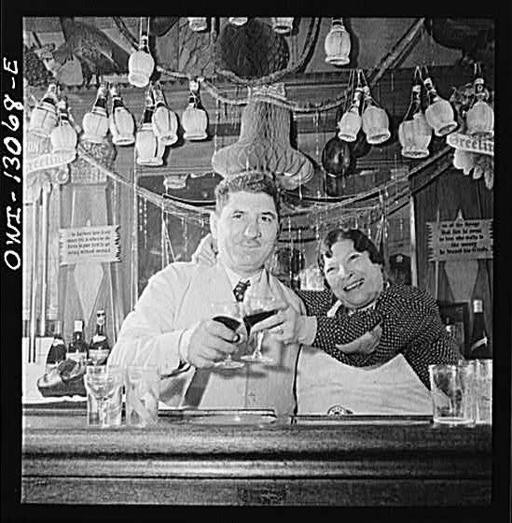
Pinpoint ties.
[233,280,252,302]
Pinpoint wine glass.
[202,298,282,370]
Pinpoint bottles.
[468,296,489,361]
[44,310,111,372]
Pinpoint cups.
[427,357,492,428]
[83,363,160,429]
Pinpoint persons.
[106,170,311,416]
[191,229,464,415]
